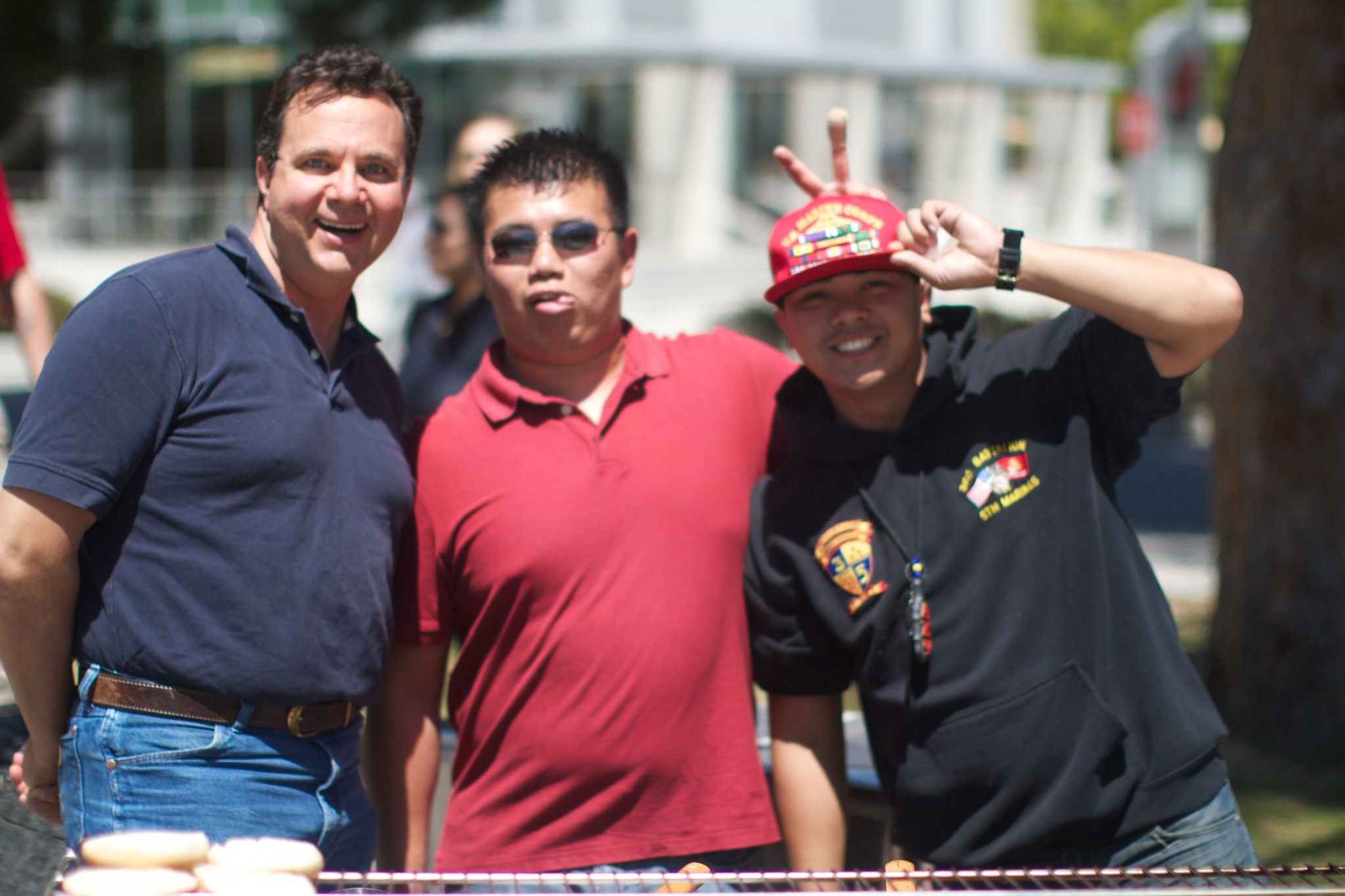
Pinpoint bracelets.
[995,228,1025,290]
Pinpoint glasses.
[473,218,622,259]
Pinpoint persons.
[0,45,423,895]
[739,194,1263,886]
[365,111,883,892]
[0,112,529,544]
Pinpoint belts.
[95,675,360,738]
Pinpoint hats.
[764,194,917,309]
